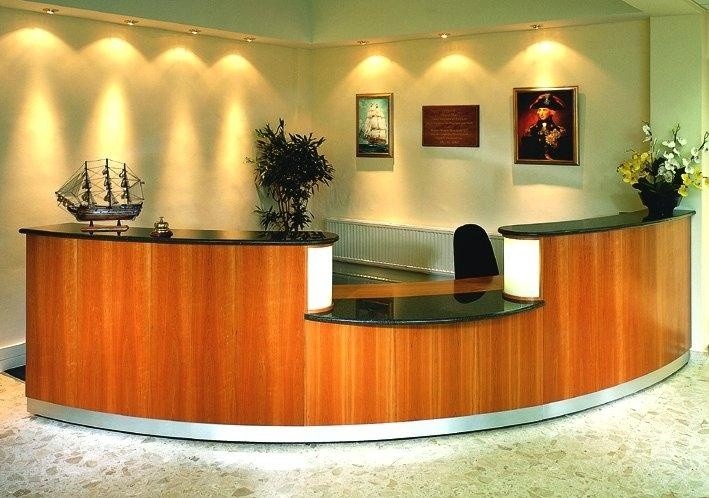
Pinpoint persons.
[521,93,572,160]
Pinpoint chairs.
[452,222,500,280]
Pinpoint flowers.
[618,119,709,197]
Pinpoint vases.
[637,190,683,218]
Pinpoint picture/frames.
[353,93,395,159]
[513,87,581,166]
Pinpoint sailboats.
[362,101,388,146]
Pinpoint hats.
[530,94,566,111]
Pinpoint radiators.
[325,216,504,278]
